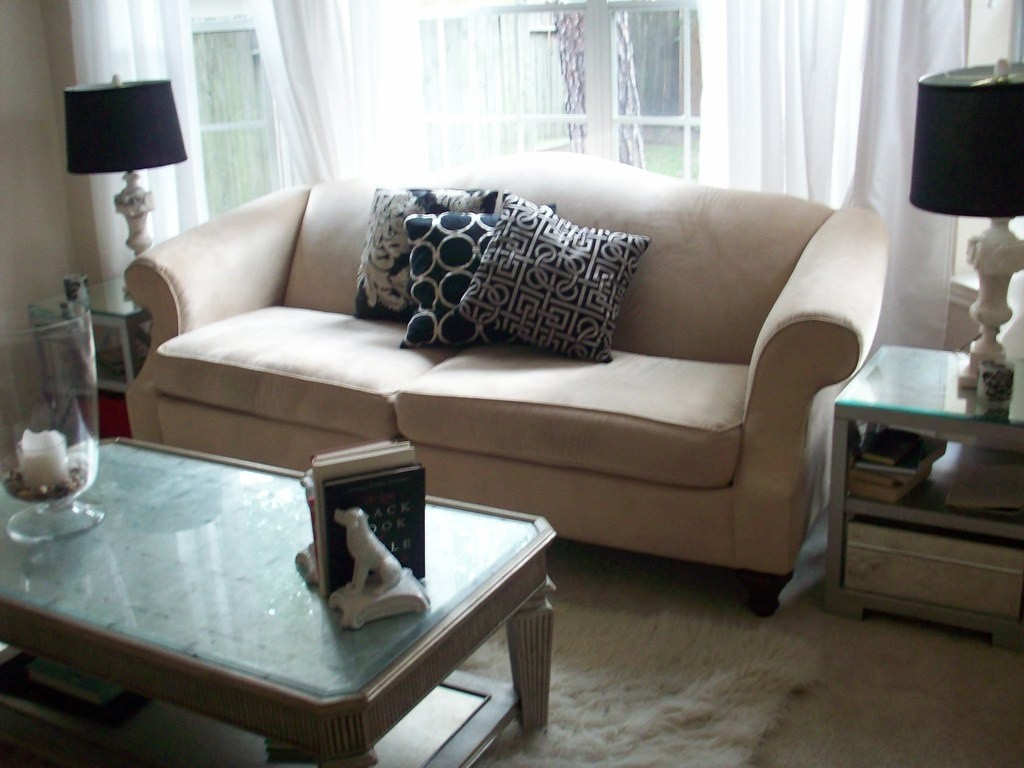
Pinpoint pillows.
[353,189,499,322]
[399,204,557,348]
[461,193,651,363]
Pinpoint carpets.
[462,605,830,768]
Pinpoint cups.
[975,359,1015,403]
[64,273,90,304]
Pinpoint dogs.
[334,507,402,597]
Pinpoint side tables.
[826,345,1024,649]
[28,277,153,393]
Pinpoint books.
[311,441,426,597]
[845,427,947,524]
[28,656,122,705]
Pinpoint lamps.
[909,65,1023,387]
[63,74,188,296]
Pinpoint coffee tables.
[0,437,559,768]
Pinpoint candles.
[20,428,66,486]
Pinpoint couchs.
[127,154,889,617]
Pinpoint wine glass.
[0,305,106,544]
